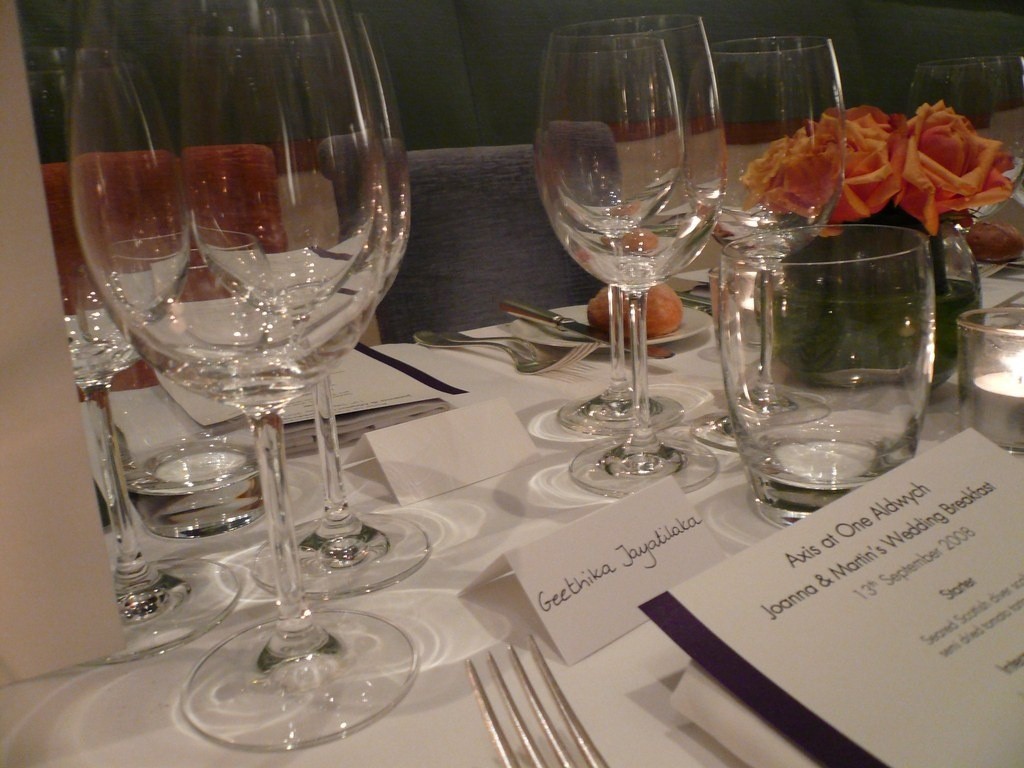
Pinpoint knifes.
[501,299,674,359]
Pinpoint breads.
[584,279,684,338]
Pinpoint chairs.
[39,145,287,399]
[313,120,632,349]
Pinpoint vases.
[753,200,984,391]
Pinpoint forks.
[415,332,601,375]
[466,634,610,768]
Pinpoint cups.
[718,226,935,528]
[957,306,1024,454]
[105,362,281,542]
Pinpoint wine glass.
[72,0,418,753]
[692,37,848,452]
[181,9,410,599]
[552,38,687,434]
[535,15,728,497]
[24,46,239,665]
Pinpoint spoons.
[437,330,610,361]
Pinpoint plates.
[512,306,713,346]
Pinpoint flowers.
[739,99,1016,241]
